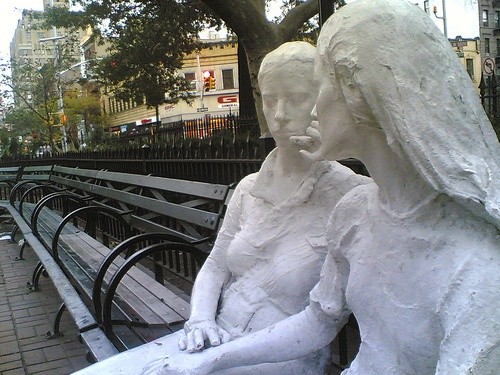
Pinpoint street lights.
[53,56,119,155]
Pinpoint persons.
[71,42,374,374]
[141,0,500,375]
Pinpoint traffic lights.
[204,77,210,92]
[210,76,215,90]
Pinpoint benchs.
[0,164,349,375]
